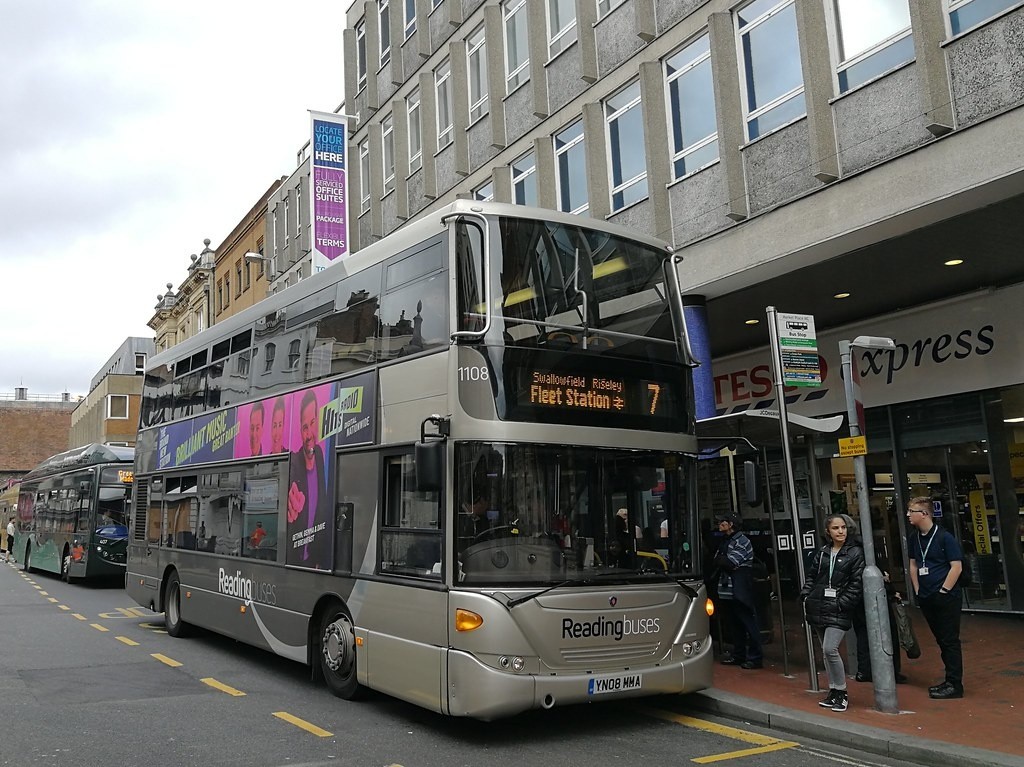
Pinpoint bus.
[124,196,715,722]
[12,443,137,582]
[0,483,22,553]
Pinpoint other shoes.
[894,674,908,683]
[855,672,872,681]
[3,559,9,564]
[720,656,747,664]
[741,659,763,668]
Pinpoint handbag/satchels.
[749,555,775,595]
[894,598,921,658]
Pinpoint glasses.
[906,508,930,516]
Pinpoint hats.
[715,511,743,530]
[840,513,857,535]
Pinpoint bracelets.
[942,587,951,594]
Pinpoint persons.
[800,515,908,712]
[506,506,765,671]
[97,509,113,527]
[907,497,966,699]
[4,517,18,564]
[249,390,329,572]
[198,522,210,547]
[453,484,497,559]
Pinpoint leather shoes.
[928,680,964,698]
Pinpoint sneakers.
[818,688,842,707]
[830,690,849,711]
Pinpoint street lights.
[243,250,275,295]
[836,336,896,715]
